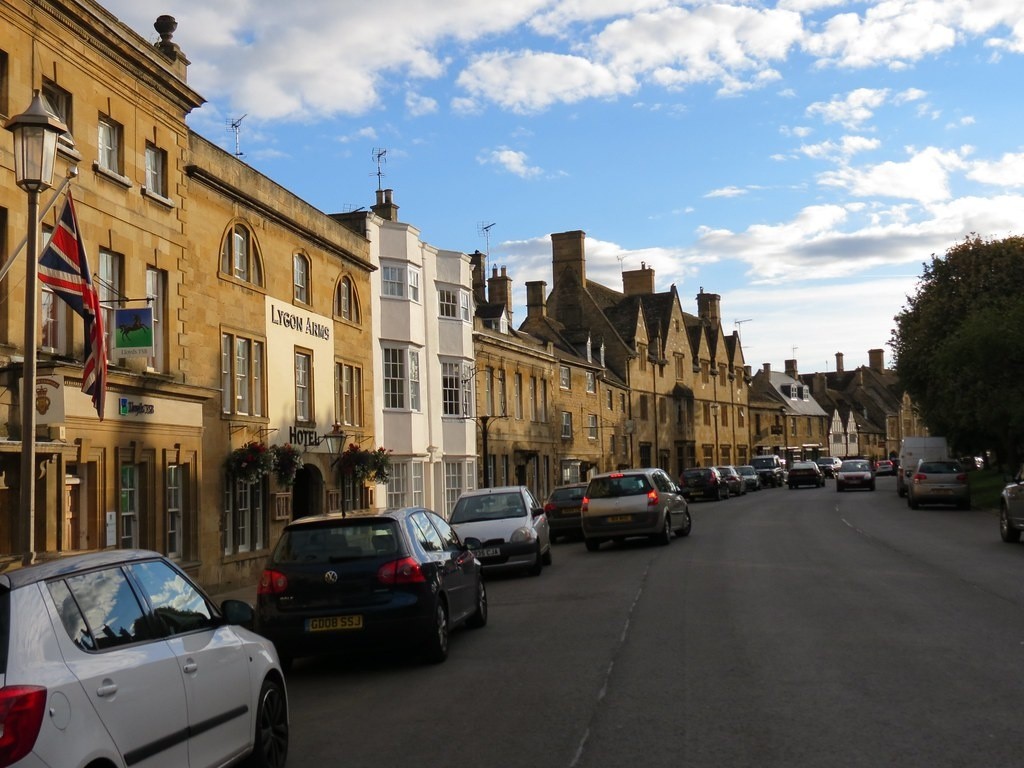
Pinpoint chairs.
[325,533,347,548]
[371,535,393,552]
[506,495,522,510]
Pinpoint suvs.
[751,455,784,488]
[817,456,842,478]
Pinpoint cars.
[448,485,553,577]
[678,466,730,501]
[999,464,1024,543]
[876,461,894,477]
[736,465,761,491]
[787,461,826,489]
[580,468,692,551]
[833,459,877,493]
[717,465,746,496]
[255,507,489,665]
[545,482,590,542]
[907,459,972,510]
[0,549,292,768]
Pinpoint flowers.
[222,441,305,485]
[337,443,395,486]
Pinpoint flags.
[38,189,107,423]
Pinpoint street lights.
[3,87,70,555]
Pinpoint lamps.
[317,419,347,457]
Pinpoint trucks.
[897,437,950,497]
